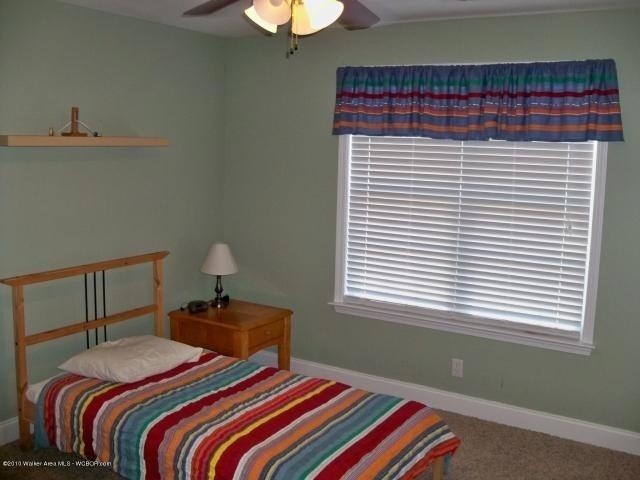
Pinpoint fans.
[179,0,383,33]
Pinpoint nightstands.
[165,295,298,374]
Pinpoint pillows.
[53,334,204,384]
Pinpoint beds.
[1,248,468,479]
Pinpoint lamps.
[242,0,347,58]
[197,238,241,310]
